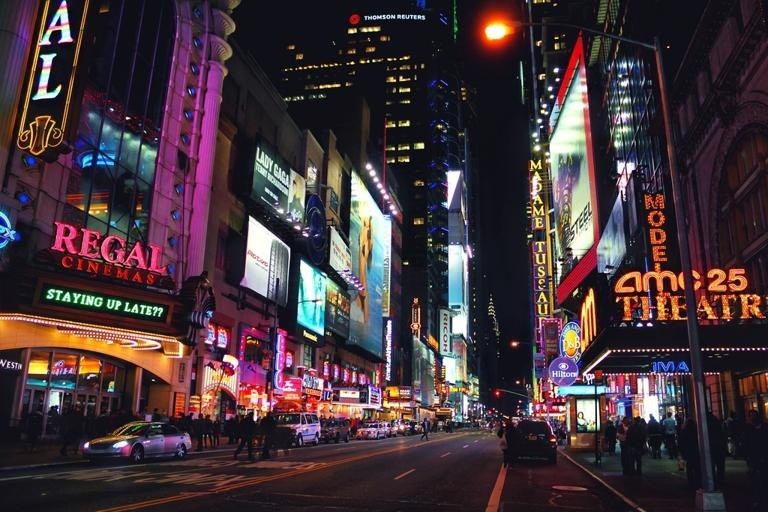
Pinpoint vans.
[506,418,559,465]
[271,411,321,446]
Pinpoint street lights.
[510,340,561,440]
[483,20,718,493]
[269,277,324,416]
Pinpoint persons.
[28,408,44,455]
[183,411,278,461]
[59,404,161,455]
[421,418,430,441]
[604,408,768,494]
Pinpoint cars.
[83,420,193,464]
[405,420,424,434]
[390,418,408,436]
[379,422,398,438]
[356,423,388,440]
[318,419,351,443]
[429,417,486,432]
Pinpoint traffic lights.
[495,389,500,397]
